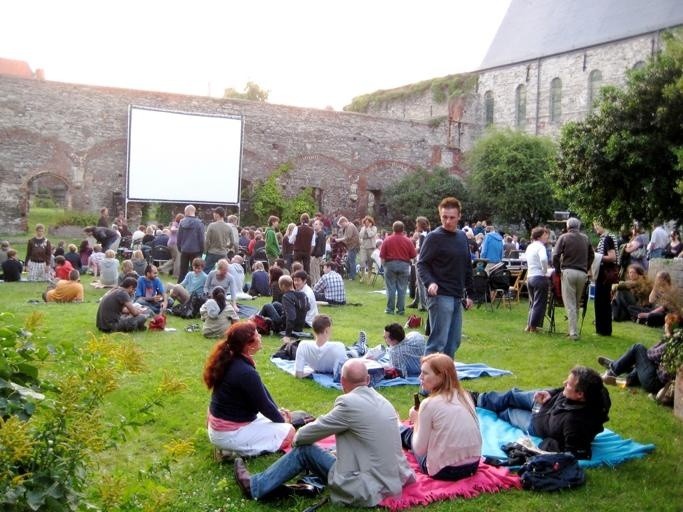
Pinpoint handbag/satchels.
[403,314,423,330]
[180,291,203,321]
[252,314,274,336]
[656,380,674,404]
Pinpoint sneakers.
[597,356,614,369]
[599,369,618,386]
[395,310,404,315]
[524,327,543,333]
[385,309,395,315]
[356,330,368,356]
[566,333,579,340]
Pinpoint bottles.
[155,287,163,302]
[411,391,420,409]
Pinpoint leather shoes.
[278,483,316,499]
[233,457,252,499]
[405,301,418,308]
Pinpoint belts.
[384,258,412,264]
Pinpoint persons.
[598,313,682,393]
[234,359,415,507]
[83,205,431,339]
[417,197,476,397]
[401,352,482,482]
[473,366,612,461]
[204,321,296,464]
[382,324,430,377]
[2,221,92,303]
[294,316,368,380]
[458,218,683,340]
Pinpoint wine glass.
[614,377,628,394]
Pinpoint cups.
[530,392,544,413]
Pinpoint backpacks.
[519,453,589,493]
[273,340,301,360]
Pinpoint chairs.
[251,246,267,264]
[236,246,252,280]
[151,244,170,262]
[367,256,384,289]
[469,266,528,312]
